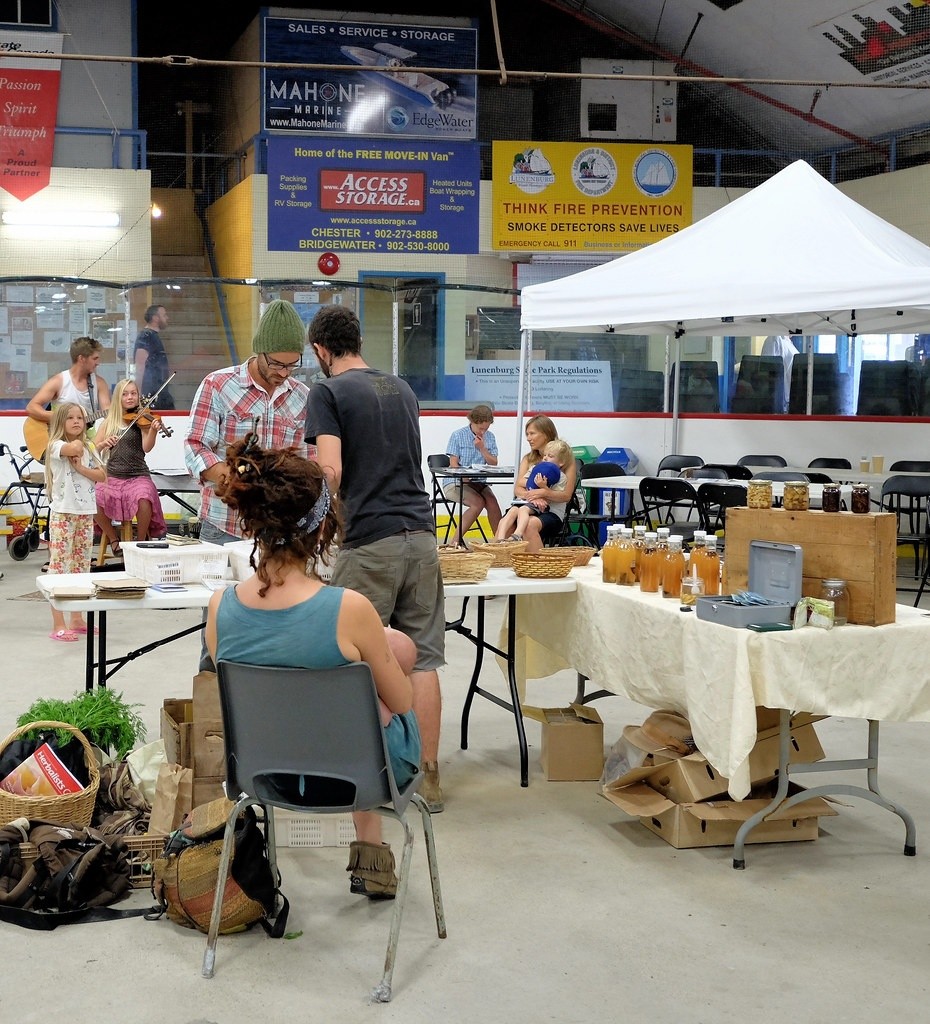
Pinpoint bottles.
[663,537,684,598]
[602,526,621,584]
[819,578,850,625]
[680,577,705,605]
[632,526,646,583]
[613,524,625,538]
[671,535,685,578]
[747,480,772,509]
[656,528,670,586]
[640,532,659,592]
[616,529,636,586]
[689,531,706,578]
[783,480,810,512]
[851,484,871,513]
[702,535,720,595]
[821,483,841,512]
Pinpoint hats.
[526,462,560,490]
[252,299,306,355]
[624,710,697,759]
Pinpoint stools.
[94,500,153,567]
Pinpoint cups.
[872,455,884,475]
[859,460,870,473]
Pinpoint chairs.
[426,454,490,548]
[199,657,454,1004]
[537,453,930,611]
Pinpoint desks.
[148,474,204,516]
[433,464,523,549]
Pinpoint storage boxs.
[0,490,14,551]
[220,780,358,848]
[224,539,261,579]
[605,706,845,851]
[118,538,233,584]
[722,506,899,627]
[158,697,193,771]
[522,698,603,784]
[317,544,338,581]
[693,538,804,625]
[162,519,191,539]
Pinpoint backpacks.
[148,795,287,938]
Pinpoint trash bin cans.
[563,445,601,547]
[593,447,640,551]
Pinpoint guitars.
[22,407,113,465]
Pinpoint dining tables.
[578,472,854,522]
[747,462,930,594]
[36,564,578,789]
[496,546,930,871]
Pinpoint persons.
[45,402,106,643]
[183,299,319,547]
[26,337,111,572]
[133,305,175,410]
[94,379,163,556]
[303,305,447,814]
[493,414,576,551]
[442,405,502,549]
[204,431,417,900]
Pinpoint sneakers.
[345,839,397,897]
[415,760,445,812]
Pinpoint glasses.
[263,353,302,370]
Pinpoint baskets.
[0,721,100,830]
[434,538,596,579]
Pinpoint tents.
[511,159,930,523]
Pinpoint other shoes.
[110,538,123,557]
[41,561,50,573]
[90,558,98,566]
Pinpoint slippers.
[48,629,79,641]
[70,623,100,636]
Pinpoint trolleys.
[0,443,52,561]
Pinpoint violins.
[122,408,175,440]
